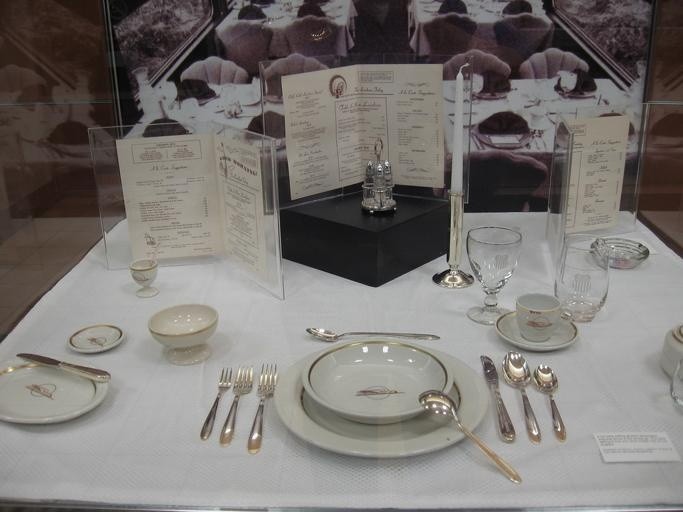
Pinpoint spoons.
[502,350,542,445]
[305,326,441,344]
[532,363,567,444]
[419,390,523,485]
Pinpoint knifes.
[16,351,111,383]
[477,352,517,444]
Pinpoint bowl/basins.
[301,336,455,422]
[147,303,218,366]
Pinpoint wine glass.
[463,225,522,326]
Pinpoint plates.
[0,361,109,425]
[67,322,124,351]
[443,79,596,150]
[273,339,490,459]
[494,310,579,353]
[154,80,262,134]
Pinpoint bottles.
[130,64,162,123]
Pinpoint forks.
[246,362,277,455]
[219,363,254,447]
[198,367,234,441]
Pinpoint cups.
[129,257,163,299]
[553,233,611,323]
[511,293,574,343]
[670,360,683,405]
